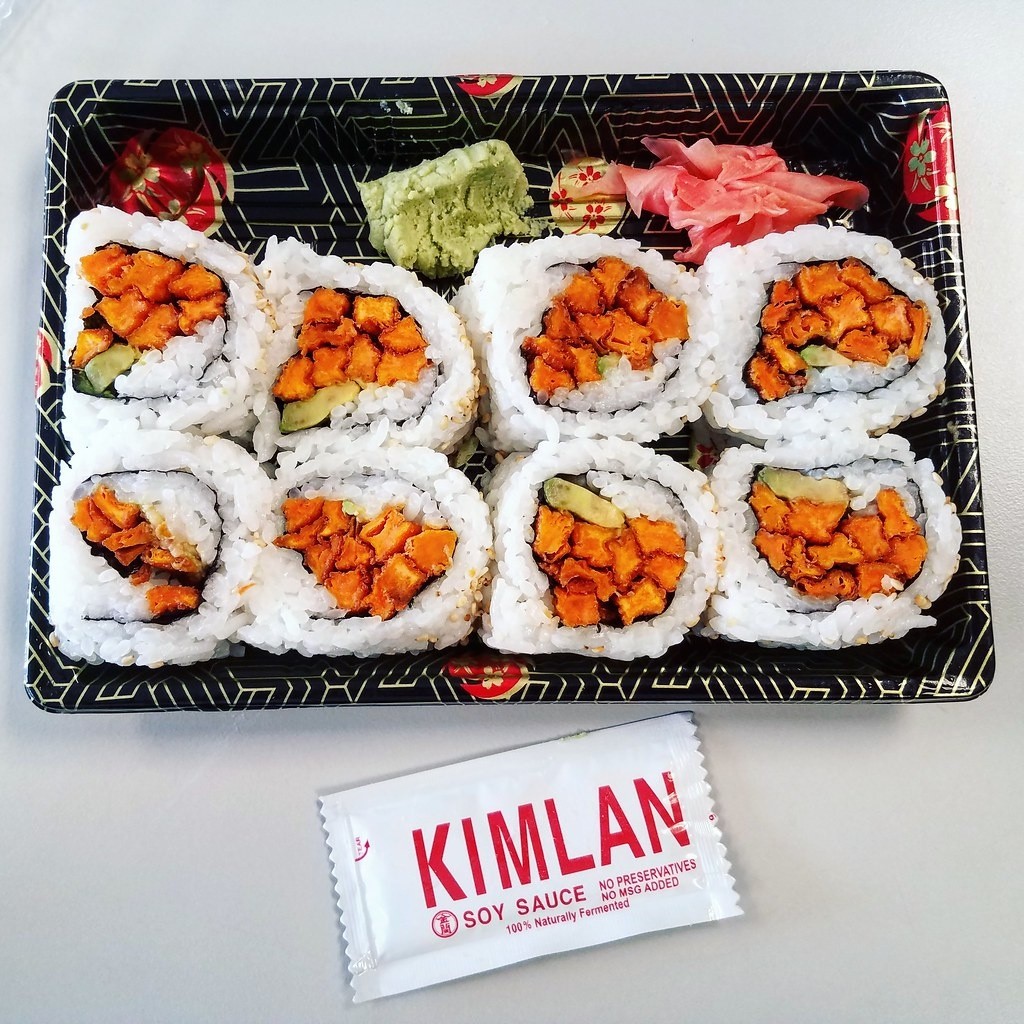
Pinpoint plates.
[26,70,995,715]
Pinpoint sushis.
[48,204,963,663]
